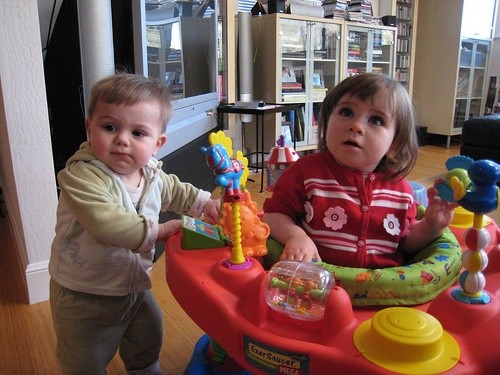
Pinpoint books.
[396,0,413,81]
[321,0,382,24]
[348,33,384,76]
[281,25,335,144]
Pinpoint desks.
[217,103,302,194]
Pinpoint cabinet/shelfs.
[146,15,211,101]
[426,37,491,148]
[245,0,420,155]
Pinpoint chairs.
[461,113,500,166]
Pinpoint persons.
[48,74,222,375]
[263,71,461,263]
